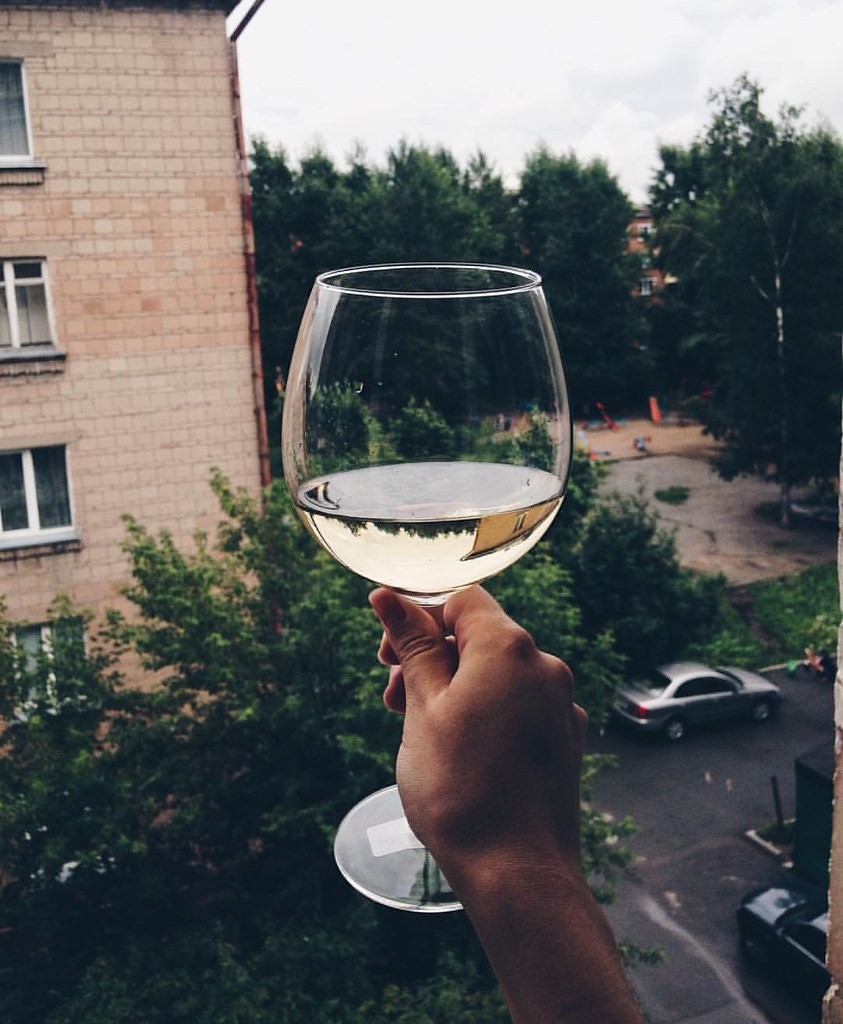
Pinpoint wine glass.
[280,260,574,915]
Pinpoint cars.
[609,654,781,741]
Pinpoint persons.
[368,583,643,1023]
[491,396,654,456]
